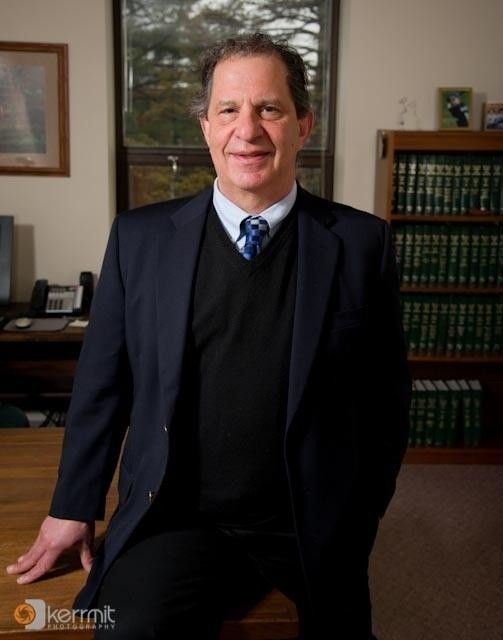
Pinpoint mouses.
[15,317,33,328]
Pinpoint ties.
[242,216,269,261]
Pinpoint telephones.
[28,279,80,319]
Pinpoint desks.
[1,426,299,638]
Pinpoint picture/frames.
[436,86,473,131]
[0,39,72,178]
[482,102,502,132]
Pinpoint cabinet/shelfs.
[372,130,503,464]
[0,303,90,412]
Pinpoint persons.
[446,92,469,126]
[6,33,411,640]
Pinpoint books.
[391,153,503,448]
